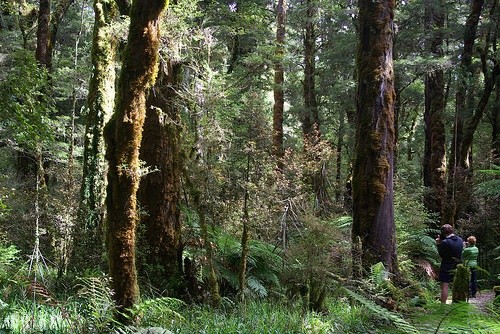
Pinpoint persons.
[463,236,479,299]
[435,223,464,304]
